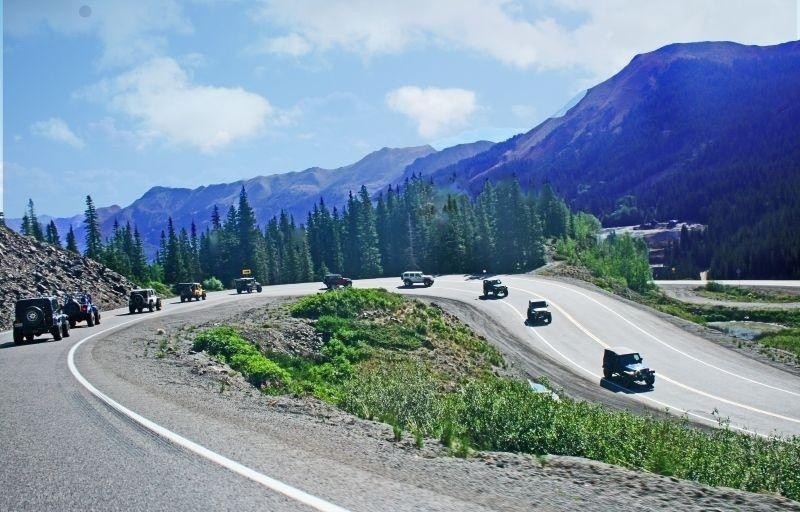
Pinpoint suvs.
[179,282,206,302]
[324,274,352,289]
[12,297,71,345]
[602,347,655,388]
[483,278,509,298]
[234,278,262,293]
[62,292,100,327]
[527,299,552,323]
[128,289,161,313]
[401,271,434,288]
